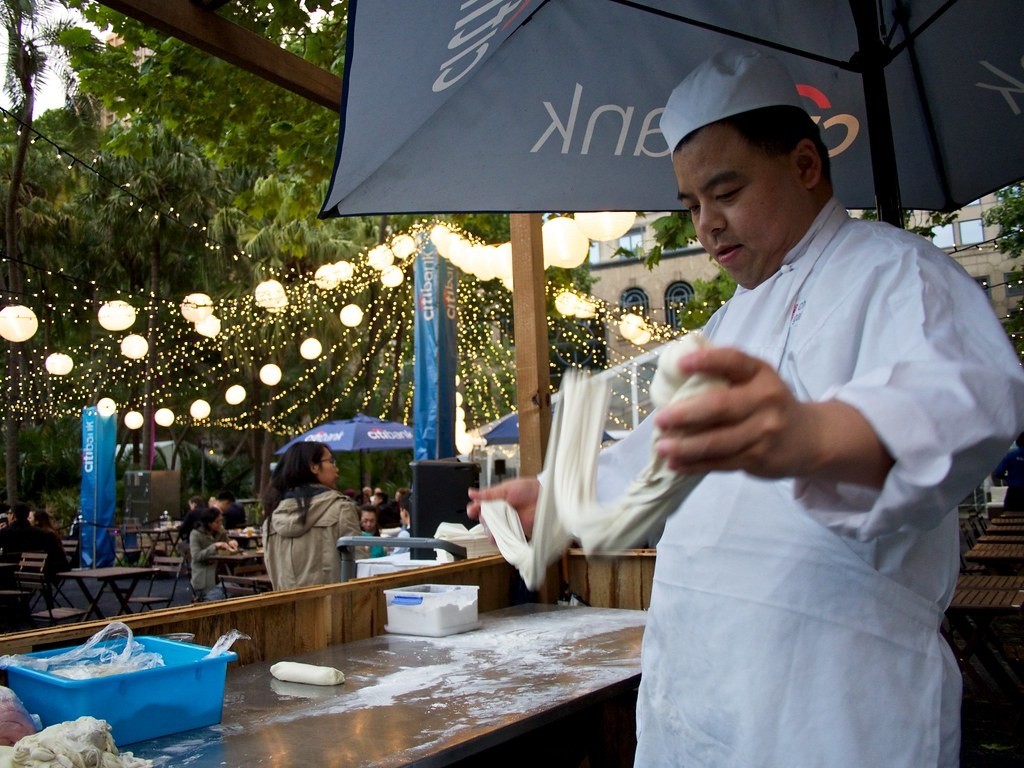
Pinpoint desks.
[56,567,161,622]
[948,511,1024,712]
[110,527,180,556]
[206,550,264,577]
[226,528,263,548]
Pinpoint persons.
[994,431,1024,511]
[176,496,207,563]
[469,45,1024,768]
[217,490,246,528]
[0,503,70,610]
[263,441,369,592]
[342,486,413,556]
[189,507,239,591]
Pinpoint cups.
[247,530,254,537]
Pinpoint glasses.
[215,520,224,525]
[321,457,336,468]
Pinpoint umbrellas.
[273,416,417,502]
[319,0,1024,231]
[480,401,619,446]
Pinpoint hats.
[660,47,805,161]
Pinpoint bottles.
[159,510,168,529]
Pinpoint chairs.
[1,525,266,625]
[939,508,1001,702]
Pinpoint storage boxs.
[0,636,239,743]
[382,584,480,632]
